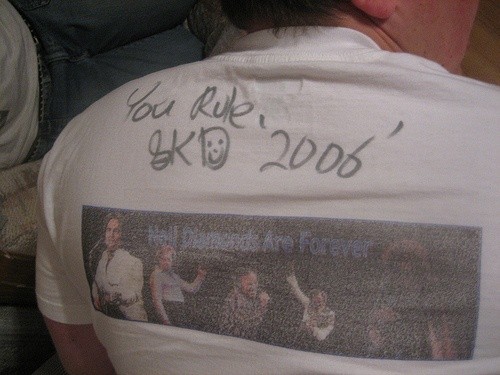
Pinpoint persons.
[91,212,481,360]
[34,0,500,375]
[0,1,39,173]
